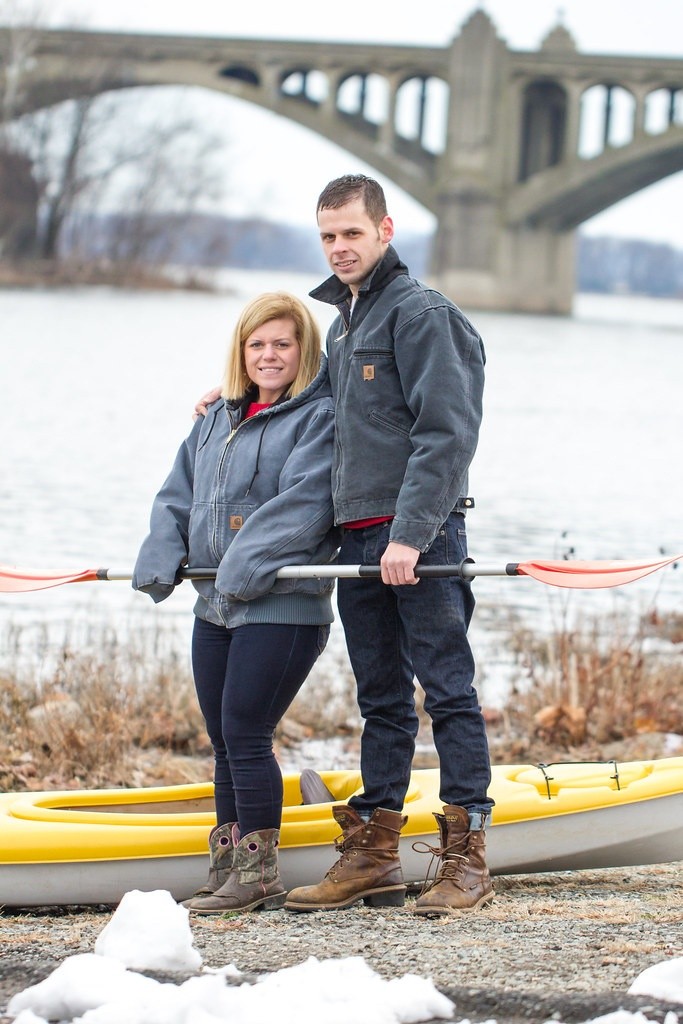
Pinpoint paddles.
[0,553,683,592]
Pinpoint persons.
[194,174,496,916]
[133,291,337,911]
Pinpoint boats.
[0,750,683,917]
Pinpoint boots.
[412,804,495,914]
[179,822,238,907]
[284,804,408,910]
[188,823,287,914]
